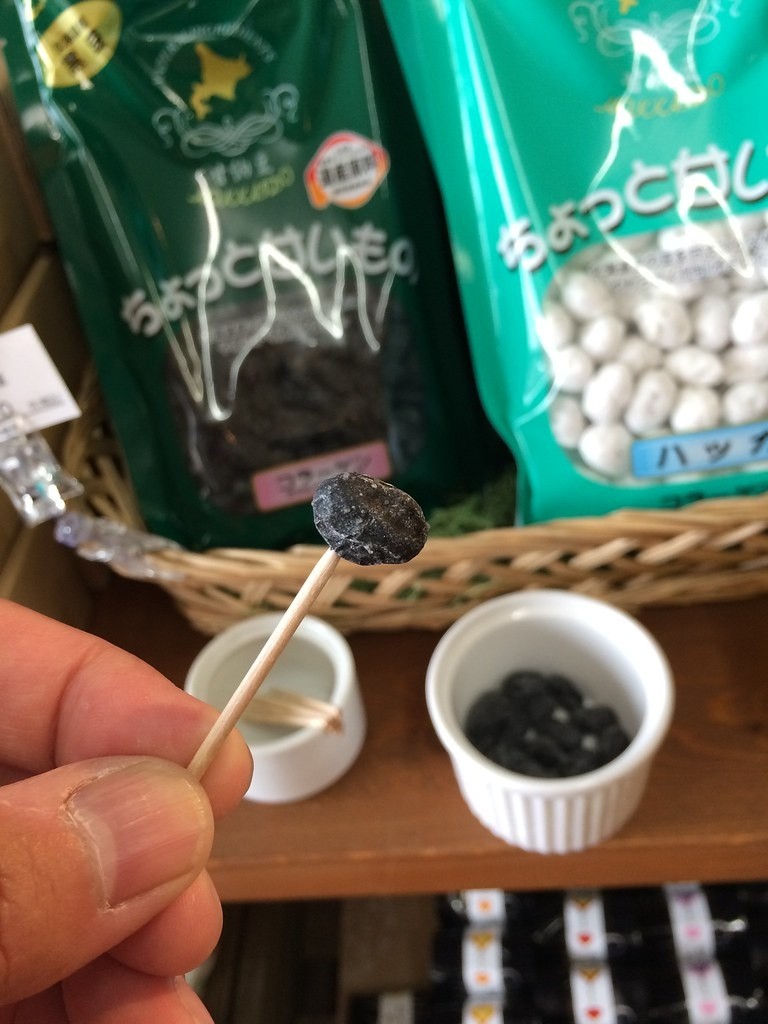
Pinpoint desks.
[86,568,767,899]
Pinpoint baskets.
[62,364,768,635]
